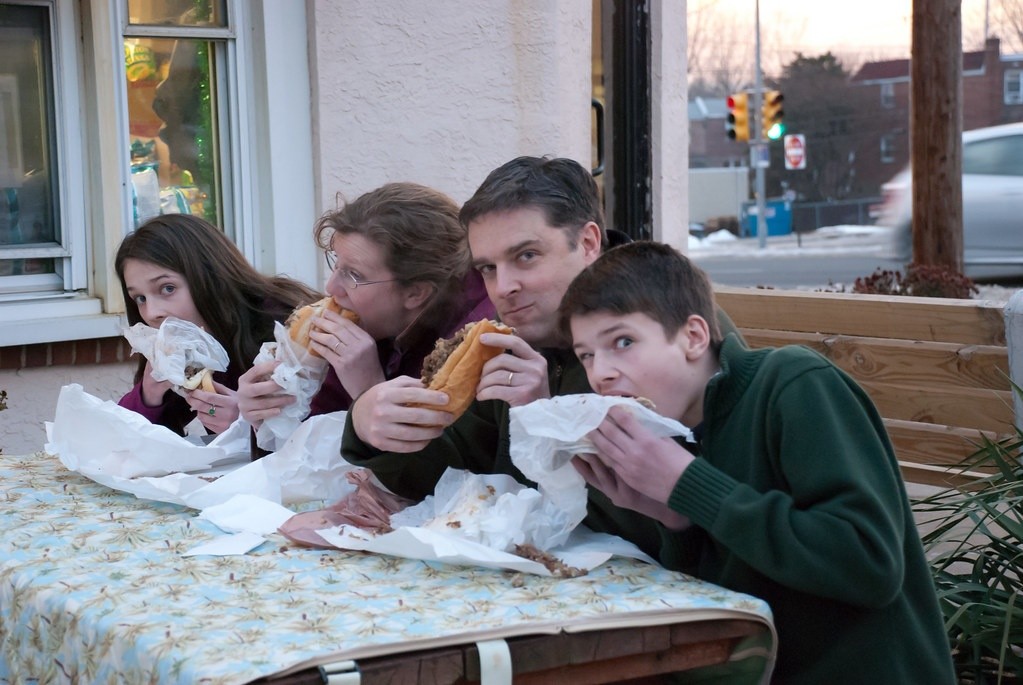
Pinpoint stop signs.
[786,136,807,168]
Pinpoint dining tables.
[0,451,780,685]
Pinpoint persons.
[114,212,328,426]
[238,182,501,460]
[565,241,959,685]
[341,156,753,557]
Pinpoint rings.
[507,372,514,385]
[334,340,340,352]
[208,404,216,416]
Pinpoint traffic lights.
[726,92,750,144]
[762,91,783,142]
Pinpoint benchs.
[710,289,1023,499]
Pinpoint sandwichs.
[180,365,217,396]
[277,294,359,386]
[407,317,520,428]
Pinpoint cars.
[879,123,1023,285]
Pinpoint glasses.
[324,249,439,290]
